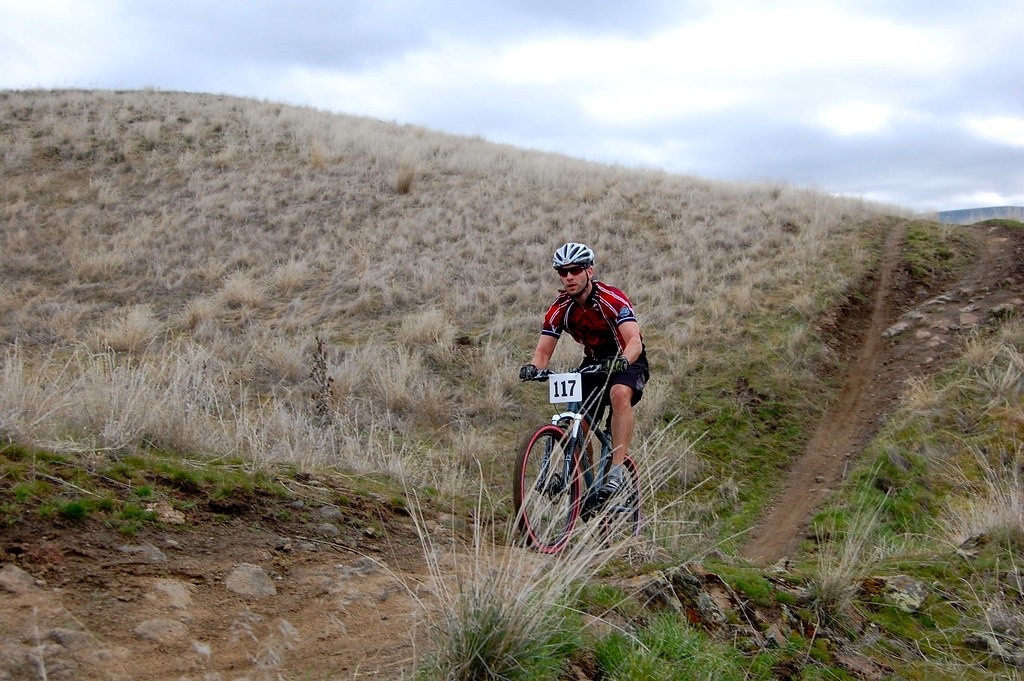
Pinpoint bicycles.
[512,362,643,559]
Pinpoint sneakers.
[598,477,621,497]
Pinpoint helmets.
[553,243,595,269]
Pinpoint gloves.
[603,355,629,374]
[519,363,538,379]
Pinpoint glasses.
[558,263,590,277]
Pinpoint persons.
[520,242,649,501]
